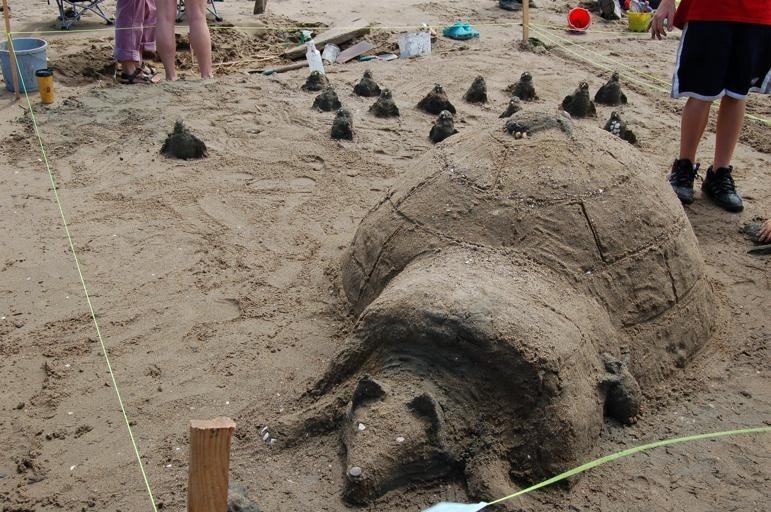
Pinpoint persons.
[756,218,771,243]
[154,1,213,82]
[115,1,162,85]
[653,1,771,211]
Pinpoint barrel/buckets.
[567,7,590,32]
[627,10,651,32]
[0,38,47,91]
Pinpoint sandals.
[120,68,155,84]
[443,22,480,40]
[141,61,157,74]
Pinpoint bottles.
[35,68,55,104]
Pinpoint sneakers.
[701,165,743,212]
[669,159,700,204]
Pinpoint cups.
[323,43,340,64]
